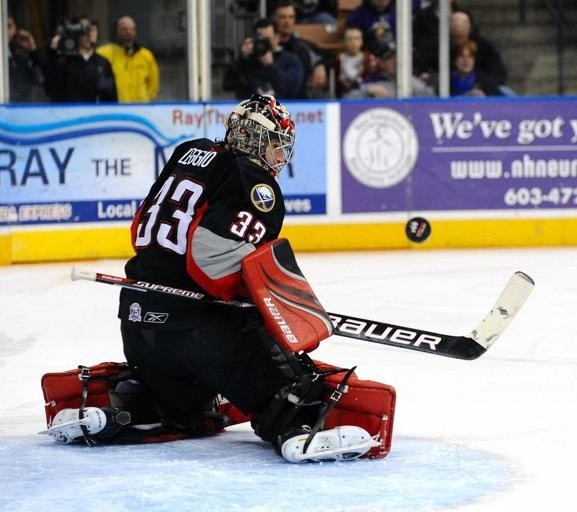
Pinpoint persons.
[37,93,382,466]
[7,10,160,103]
[222,1,507,105]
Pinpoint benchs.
[252,0,437,99]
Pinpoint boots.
[278,424,376,463]
[49,404,127,444]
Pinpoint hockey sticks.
[70,267,536,360]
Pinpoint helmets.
[226,92,297,177]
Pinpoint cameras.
[252,37,271,56]
